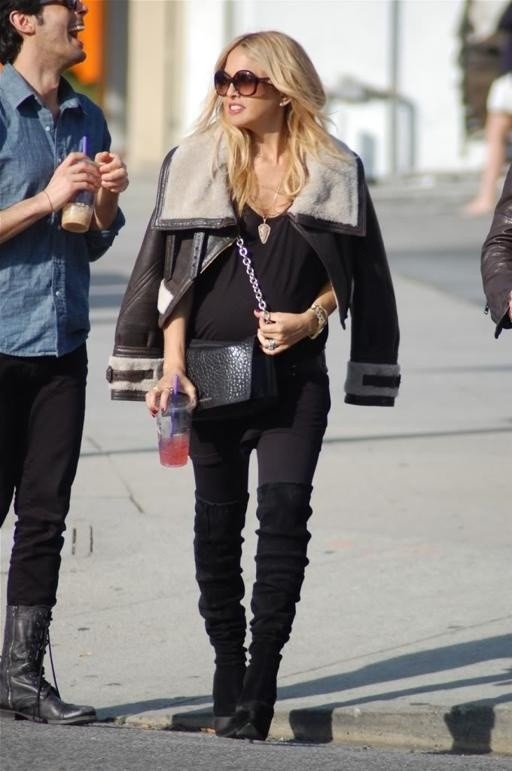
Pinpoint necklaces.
[250,147,288,249]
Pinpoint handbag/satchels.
[179,339,291,421]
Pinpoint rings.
[268,340,276,346]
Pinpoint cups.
[152,392,190,469]
[60,158,102,235]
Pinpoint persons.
[1,0,130,727]
[467,64,511,223]
[482,167,510,340]
[100,29,408,737]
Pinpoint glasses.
[42,0,78,12]
[214,69,271,97]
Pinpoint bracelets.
[312,305,329,343]
[40,187,55,222]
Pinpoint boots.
[0,604,98,724]
[193,483,313,740]
